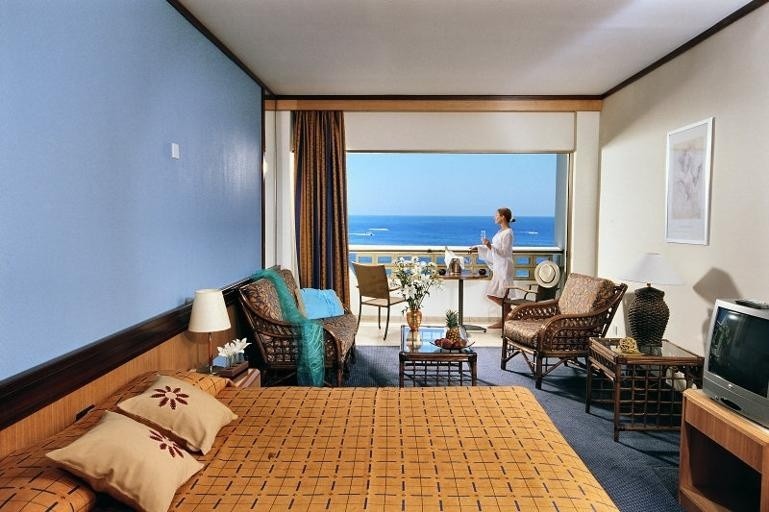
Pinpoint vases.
[391,256,437,309]
[406,309,422,332]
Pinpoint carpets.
[262,346,687,511]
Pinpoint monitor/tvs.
[702,299,769,430]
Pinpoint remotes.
[735,299,769,311]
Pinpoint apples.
[435,338,467,348]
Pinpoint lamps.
[188,289,232,374]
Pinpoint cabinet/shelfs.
[677,385,768,512]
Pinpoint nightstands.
[189,367,262,387]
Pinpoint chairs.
[351,261,409,340]
[501,281,558,337]
[500,273,628,390]
[236,270,358,386]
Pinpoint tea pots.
[448,258,462,275]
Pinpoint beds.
[0,386,620,512]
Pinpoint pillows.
[0,369,236,512]
[45,410,204,512]
[117,374,238,456]
[294,287,344,319]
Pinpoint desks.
[434,268,493,333]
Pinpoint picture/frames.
[664,116,715,246]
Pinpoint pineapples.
[445,308,459,340]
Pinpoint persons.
[477,208,515,329]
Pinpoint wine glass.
[480,230,487,246]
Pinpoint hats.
[534,260,560,288]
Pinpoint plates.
[429,339,476,350]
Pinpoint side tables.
[584,337,705,443]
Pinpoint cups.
[439,269,446,275]
[479,269,486,275]
